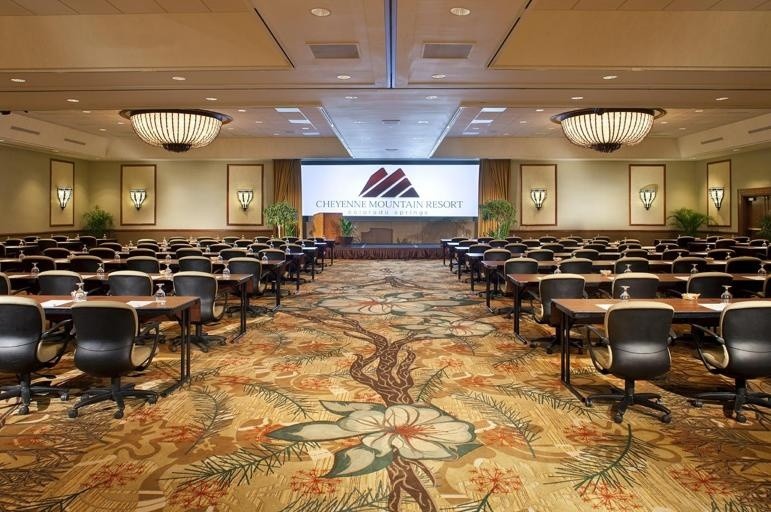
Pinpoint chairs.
[525,273,588,356]
[439,235,510,312]
[220,233,334,312]
[37,269,104,297]
[666,272,734,297]
[741,275,770,298]
[106,270,164,344]
[67,300,160,419]
[509,233,770,272]
[0,233,219,272]
[585,301,673,425]
[690,299,771,422]
[0,295,72,415]
[169,270,228,353]
[0,272,10,295]
[595,272,661,299]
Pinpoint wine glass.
[223,264,230,278]
[96,262,104,277]
[690,262,700,274]
[75,282,87,302]
[31,262,39,277]
[234,241,237,248]
[554,264,563,274]
[161,236,173,261]
[155,283,165,304]
[82,244,88,253]
[721,284,734,302]
[217,251,223,264]
[758,263,767,275]
[206,244,211,254]
[164,262,172,278]
[103,234,108,239]
[285,245,290,255]
[114,250,121,262]
[262,251,268,262]
[620,284,632,300]
[248,244,253,253]
[19,250,25,261]
[726,251,733,260]
[255,234,318,248]
[706,243,712,252]
[762,240,767,247]
[624,264,632,273]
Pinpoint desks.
[506,272,770,344]
[551,296,771,403]
[0,272,253,343]
[8,294,203,398]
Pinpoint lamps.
[640,187,655,208]
[127,110,224,154]
[707,186,724,211]
[529,188,548,210]
[128,189,146,210]
[560,108,654,154]
[236,190,252,210]
[56,186,71,210]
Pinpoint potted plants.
[336,218,353,247]
